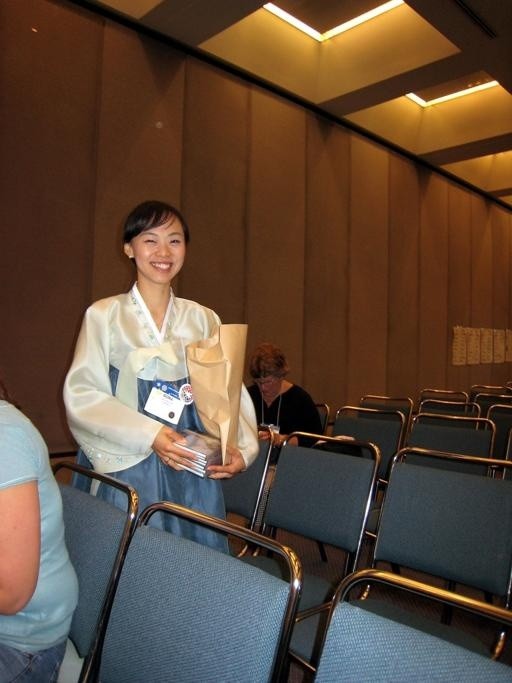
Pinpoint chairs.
[402,413,496,480]
[331,406,406,481]
[357,393,414,416]
[78,500,305,681]
[288,445,512,678]
[49,461,137,683]
[472,392,511,415]
[465,383,510,393]
[416,399,481,429]
[414,388,470,406]
[222,424,273,560]
[484,404,512,432]
[301,568,511,683]
[226,430,382,610]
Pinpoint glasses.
[252,378,273,387]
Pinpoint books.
[166,429,224,478]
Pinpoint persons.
[63,201,259,555]
[1,388,80,683]
[243,344,321,464]
